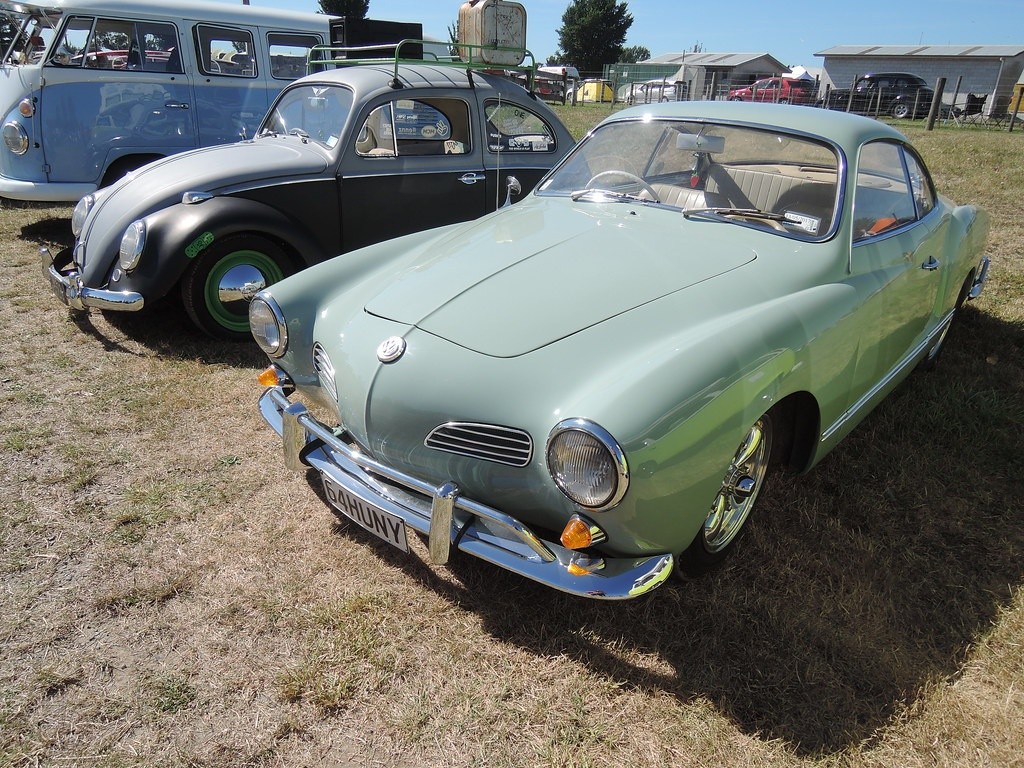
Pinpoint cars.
[248,99,992,601]
[622,78,688,105]
[517,66,618,103]
[39,40,596,342]
[60,49,240,74]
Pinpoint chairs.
[638,183,732,214]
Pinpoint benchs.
[704,164,912,235]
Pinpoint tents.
[577,83,613,102]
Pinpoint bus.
[0,0,455,204]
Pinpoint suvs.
[725,77,813,107]
[827,71,961,121]
